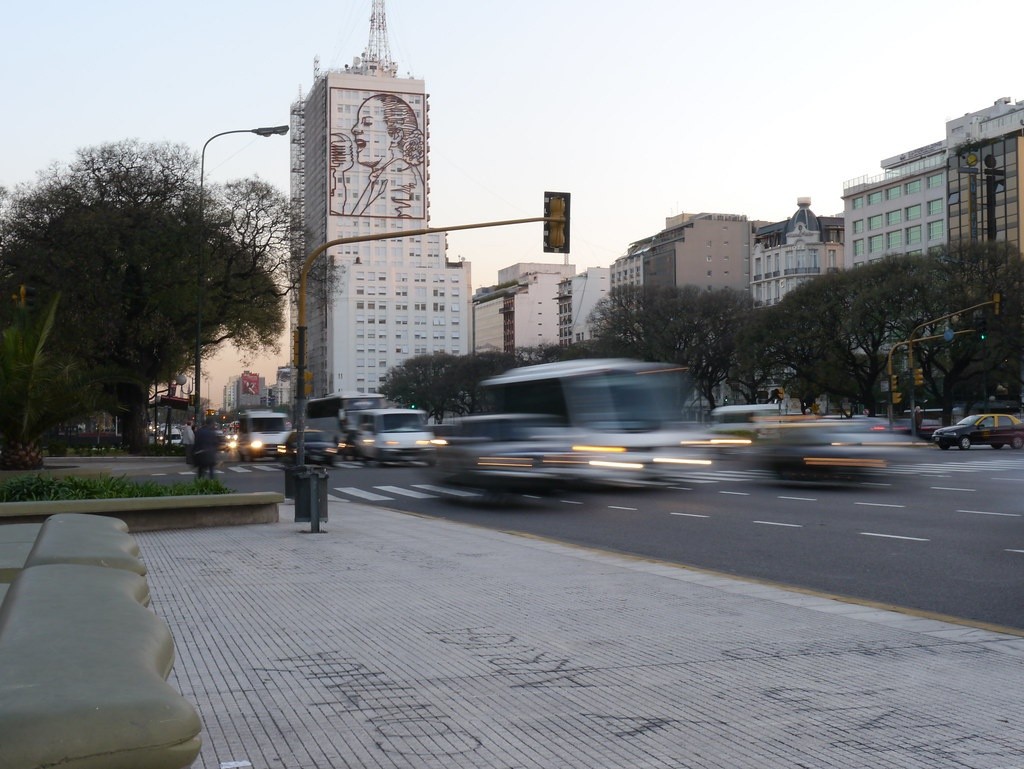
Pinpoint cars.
[917,419,944,439]
[284,428,337,468]
[164,427,182,445]
[930,414,1024,450]
[741,417,918,492]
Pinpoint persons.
[215,423,233,435]
[913,406,923,432]
[183,421,196,465]
[194,418,222,482]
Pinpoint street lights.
[190,123,292,427]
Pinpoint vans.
[439,412,596,503]
[356,408,438,467]
[237,410,292,464]
[712,400,784,453]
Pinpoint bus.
[302,393,385,459]
[477,358,694,494]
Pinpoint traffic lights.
[891,375,899,391]
[914,369,924,386]
[892,392,902,404]
[976,317,988,343]
